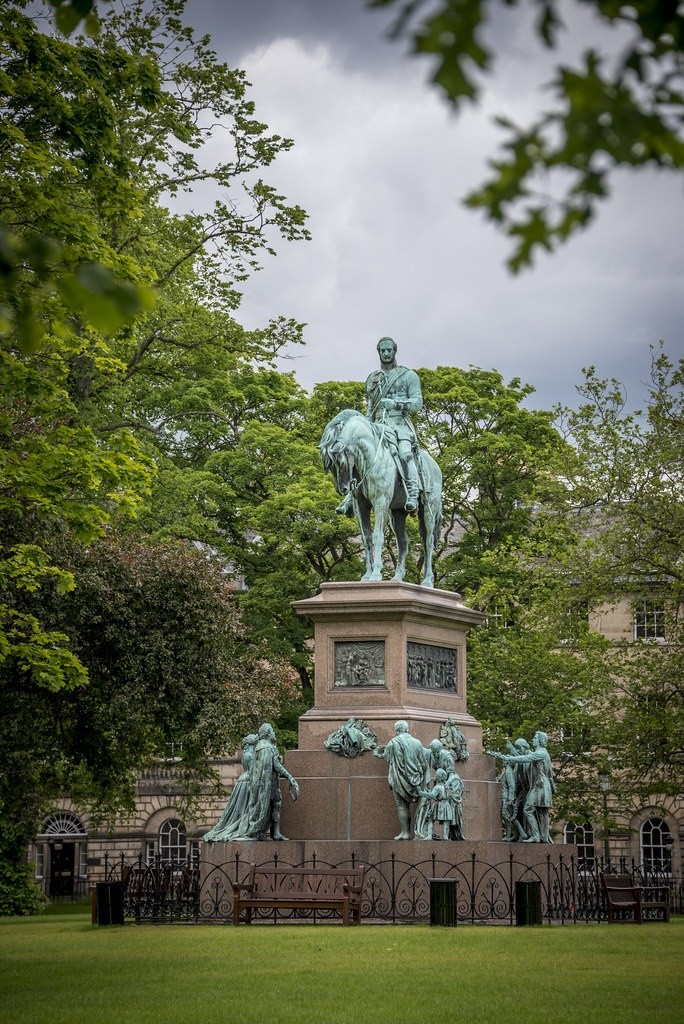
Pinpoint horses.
[316,409,444,588]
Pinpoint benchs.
[88,865,171,925]
[599,870,671,925]
[231,862,365,926]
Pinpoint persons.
[335,646,383,684]
[335,337,425,519]
[407,654,456,691]
[374,719,467,841]
[486,731,556,846]
[234,721,299,840]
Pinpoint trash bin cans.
[515,881,542,926]
[96,881,124,925]
[429,878,457,928]
